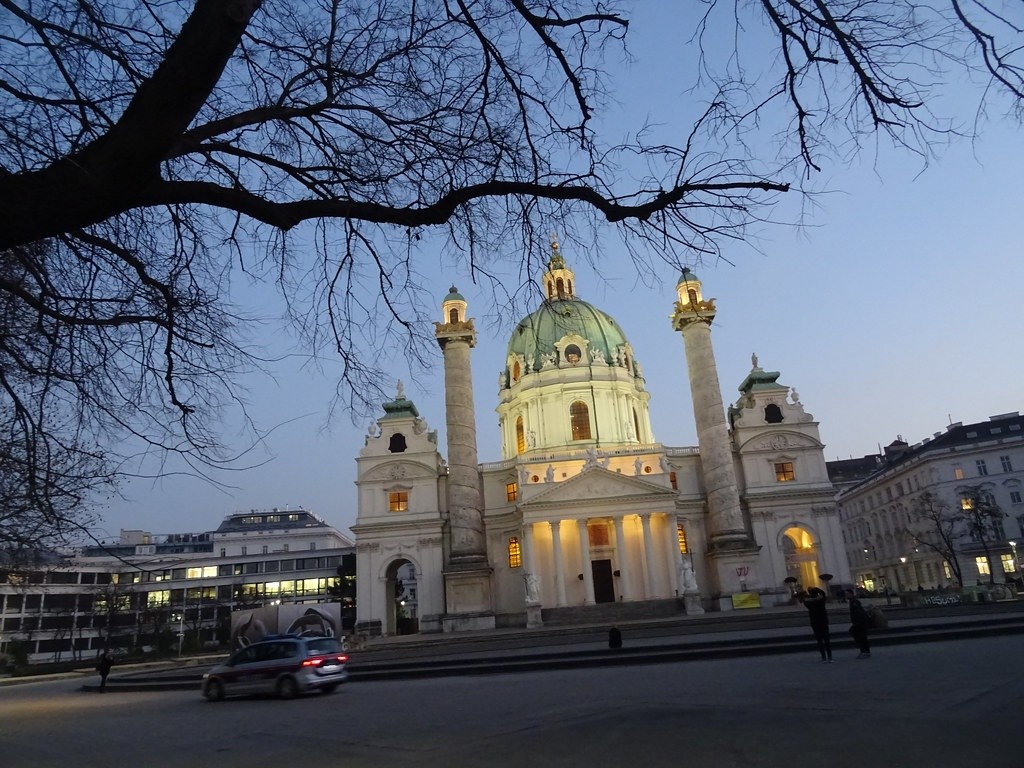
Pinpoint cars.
[201,638,348,702]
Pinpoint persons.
[100,650,114,692]
[917,583,942,590]
[235,630,250,650]
[976,578,982,585]
[846,588,870,659]
[793,587,847,606]
[803,587,835,663]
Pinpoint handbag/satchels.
[96,659,103,671]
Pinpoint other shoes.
[828,657,834,663]
[861,652,871,657]
[822,658,828,664]
